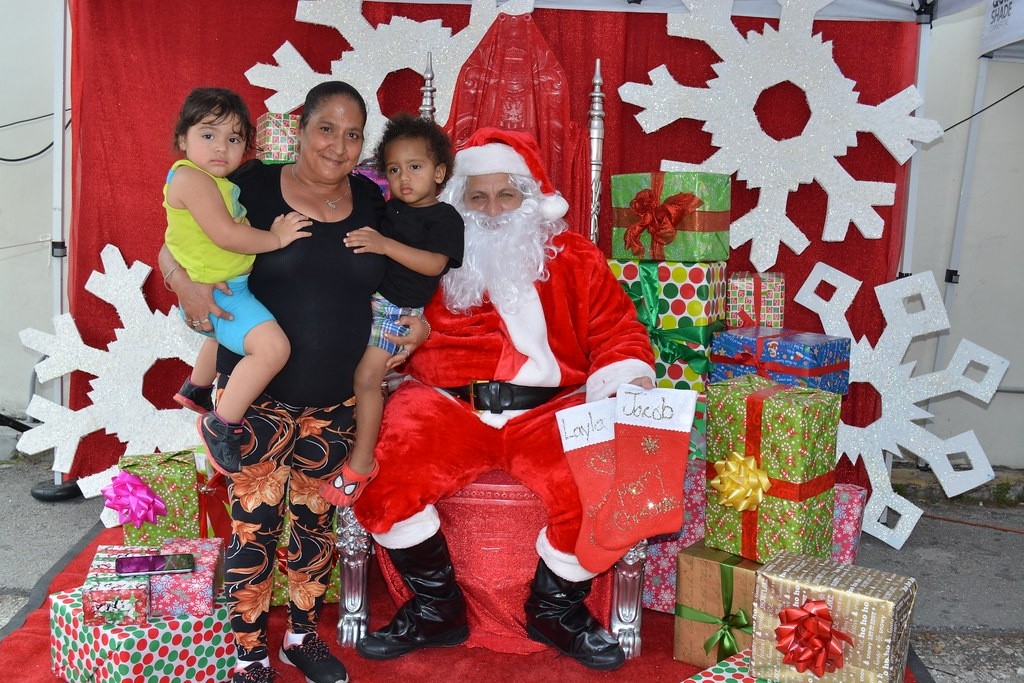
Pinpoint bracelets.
[423,319,431,340]
[164,267,177,291]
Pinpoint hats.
[452,126,569,220]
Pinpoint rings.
[200,319,210,323]
[403,349,410,356]
[193,320,200,326]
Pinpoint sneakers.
[278,629,349,683]
[230,663,277,683]
[197,410,245,478]
[172,375,214,415]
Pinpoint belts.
[447,380,573,414]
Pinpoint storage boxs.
[254,112,391,202]
[605,171,919,683]
[47,447,341,683]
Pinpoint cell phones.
[116,553,195,577]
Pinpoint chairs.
[335,11,650,660]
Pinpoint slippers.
[318,455,379,507]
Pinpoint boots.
[356,528,469,660]
[523,557,625,670]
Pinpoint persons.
[165,87,313,476]
[318,118,465,507]
[157,81,431,683]
[352,126,657,671]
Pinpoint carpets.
[0,520,935,683]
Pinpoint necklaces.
[292,163,349,210]
